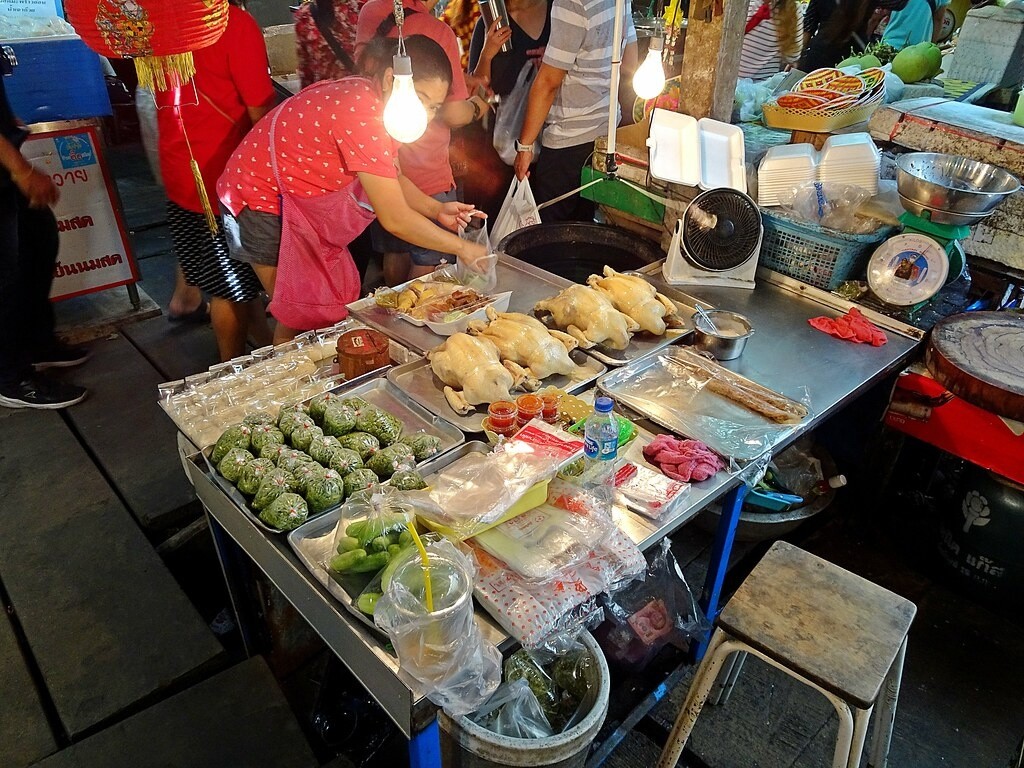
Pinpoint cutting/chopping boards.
[925,311,1024,423]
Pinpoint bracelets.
[11,161,34,181]
[471,101,480,120]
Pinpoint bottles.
[582,397,619,494]
[803,474,848,507]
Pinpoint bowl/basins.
[691,308,755,361]
[894,152,1022,214]
[757,132,881,207]
[401,284,513,336]
[646,108,748,195]
[696,494,836,541]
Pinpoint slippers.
[167,298,210,323]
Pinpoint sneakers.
[0,366,88,410]
[26,334,93,372]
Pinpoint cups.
[487,392,558,438]
[478,0,515,54]
[388,549,473,684]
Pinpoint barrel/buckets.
[497,220,666,287]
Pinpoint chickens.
[425,334,514,415]
[468,306,579,391]
[535,283,640,351]
[587,265,684,338]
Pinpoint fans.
[662,186,766,290]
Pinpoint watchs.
[514,139,535,153]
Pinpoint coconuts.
[837,42,943,85]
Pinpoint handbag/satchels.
[490,173,543,252]
[455,210,498,293]
[493,57,548,167]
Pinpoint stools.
[655,539,920,768]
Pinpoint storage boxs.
[946,5,1024,88]
[869,97,1024,272]
[882,358,1024,485]
[758,205,893,293]
[0,34,114,125]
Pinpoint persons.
[0,72,94,409]
[738,0,952,83]
[215,34,489,344]
[294,0,638,295]
[136,0,276,359]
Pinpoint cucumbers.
[331,515,417,572]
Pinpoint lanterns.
[63,0,229,92]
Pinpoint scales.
[864,152,1022,313]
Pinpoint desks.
[157,222,918,768]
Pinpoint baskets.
[757,204,894,291]
[761,98,884,133]
